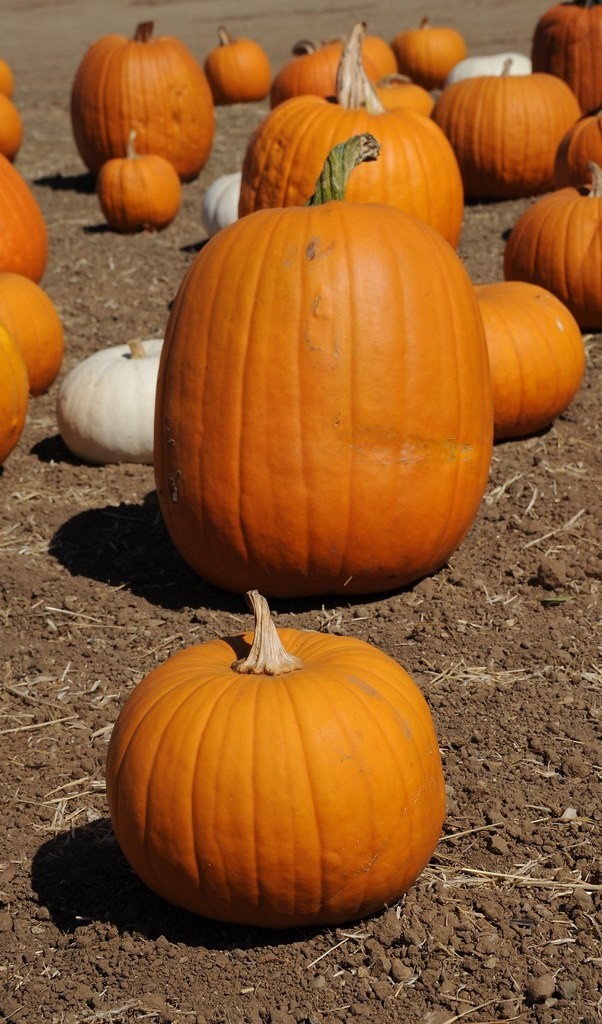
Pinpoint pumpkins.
[104,591,447,926]
[0,0,602,471]
[152,131,491,597]
